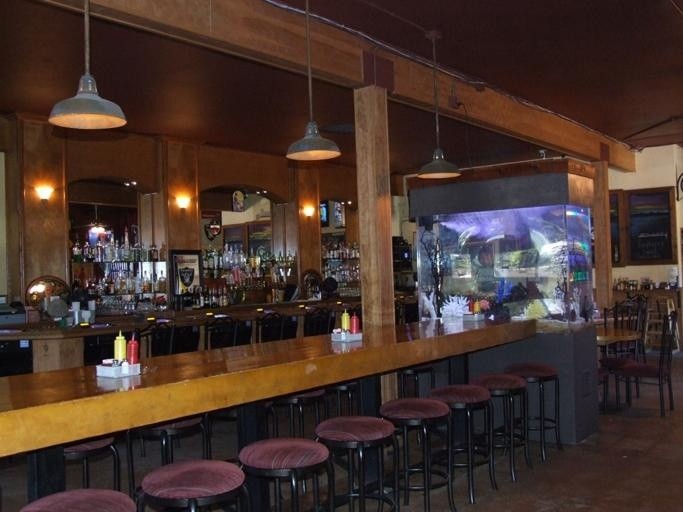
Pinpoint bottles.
[324,240,359,282]
[341,309,360,341]
[613,278,638,291]
[183,243,295,310]
[86,281,97,310]
[112,361,119,376]
[121,359,129,374]
[114,330,127,365]
[469,298,481,313]
[71,231,166,295]
[71,278,81,310]
[127,335,139,364]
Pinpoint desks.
[596,328,644,412]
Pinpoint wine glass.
[100,297,166,314]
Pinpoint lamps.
[49,0,127,130]
[285,3,341,161]
[417,31,461,180]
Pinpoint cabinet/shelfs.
[324,256,361,297]
[623,185,677,265]
[588,189,625,268]
[612,289,682,350]
[645,296,680,349]
[72,259,172,314]
[205,263,295,304]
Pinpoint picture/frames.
[170,248,204,298]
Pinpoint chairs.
[621,311,677,417]
[137,296,406,359]
[603,295,648,410]
